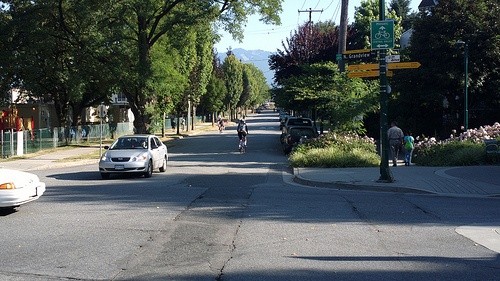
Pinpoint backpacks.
[405,141,413,150]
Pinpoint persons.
[403,130,414,166]
[237,119,249,150]
[387,121,404,166]
[142,117,185,136]
[218,118,225,132]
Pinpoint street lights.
[454,41,470,129]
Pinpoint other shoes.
[405,163,410,166]
[393,164,398,167]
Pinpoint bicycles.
[219,125,224,133]
[237,130,249,154]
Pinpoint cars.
[279,109,319,156]
[98,134,169,177]
[256,104,268,113]
[0,168,46,208]
